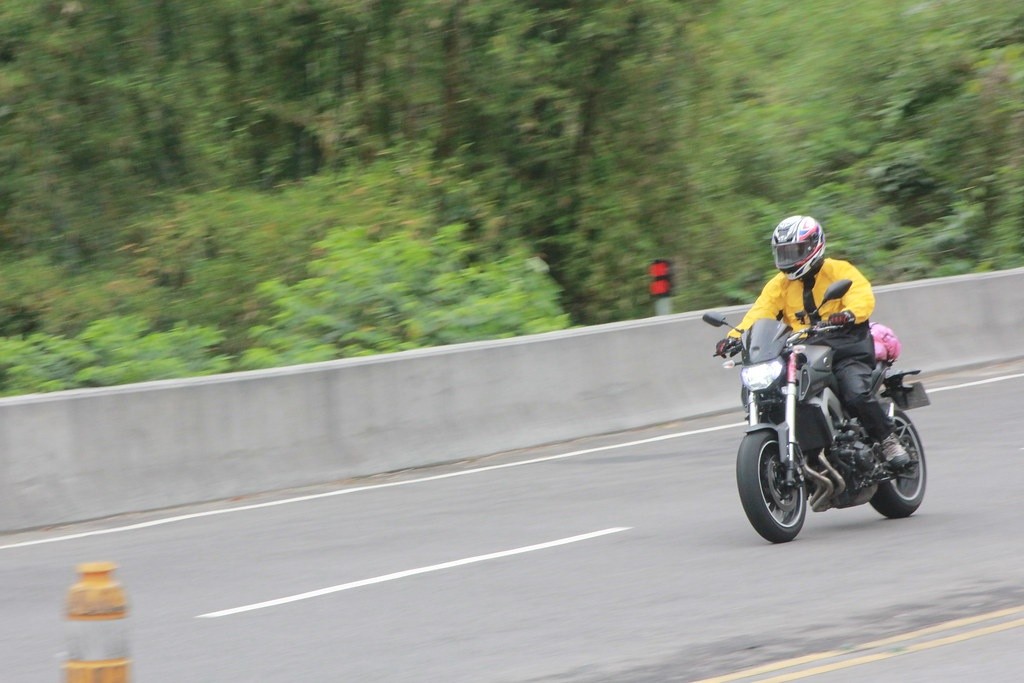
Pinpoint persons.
[715,215,911,469]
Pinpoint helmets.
[770,214,828,281]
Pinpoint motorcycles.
[702,278,931,544]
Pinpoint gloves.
[827,309,856,328]
[715,336,743,358]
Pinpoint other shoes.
[880,433,911,469]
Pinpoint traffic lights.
[646,259,674,298]
